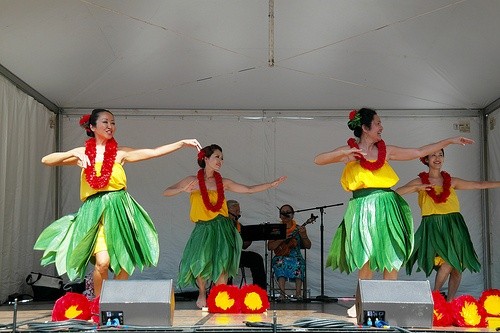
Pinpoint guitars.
[275,212,318,258]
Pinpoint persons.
[159,143,287,308]
[313,107,474,317]
[395,147,500,302]
[267,204,311,301]
[40,108,202,299]
[226,199,268,290]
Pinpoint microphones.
[282,213,291,219]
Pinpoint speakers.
[356,278,434,329]
[99,279,175,326]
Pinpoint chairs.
[271,248,306,302]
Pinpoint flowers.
[80,113,91,133]
[52,290,91,324]
[420,156,427,164]
[238,284,270,314]
[478,289,500,317]
[206,283,237,314]
[431,290,452,327]
[197,150,205,160]
[90,296,100,322]
[452,295,484,328]
[346,108,363,128]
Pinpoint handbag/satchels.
[26,271,64,303]
